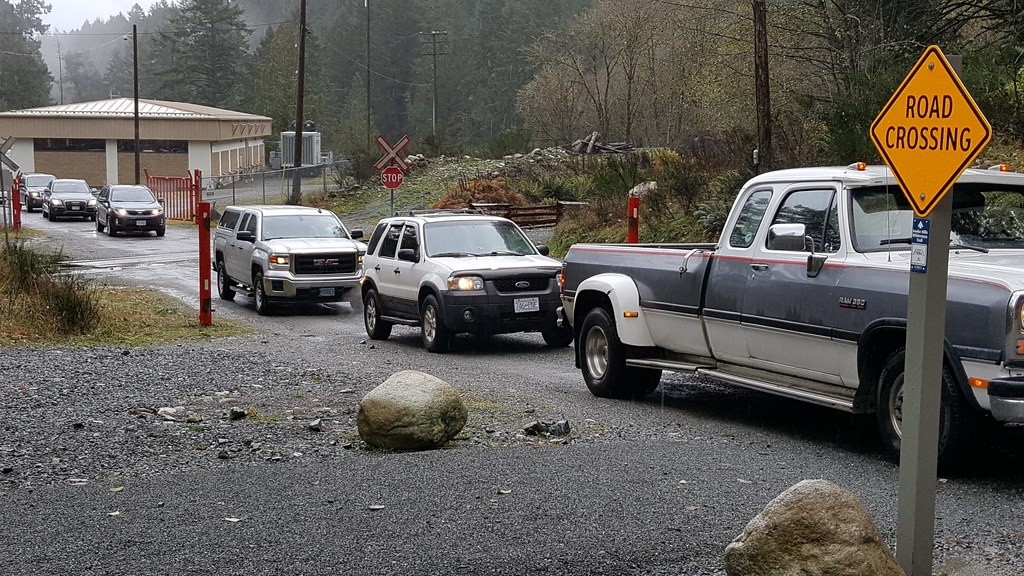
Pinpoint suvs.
[359,209,575,354]
[95,183,166,237]
[211,205,368,317]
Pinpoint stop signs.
[382,166,403,189]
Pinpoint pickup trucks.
[559,162,1024,471]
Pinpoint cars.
[19,172,57,213]
[42,179,97,221]
